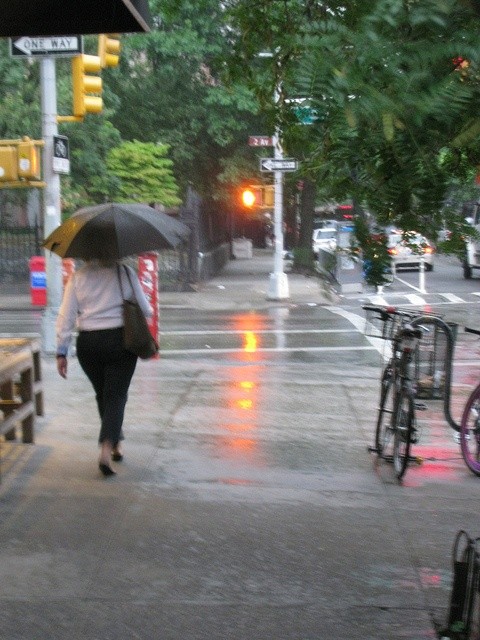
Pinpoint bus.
[315,205,356,221]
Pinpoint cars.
[312,228,337,255]
[387,231,433,274]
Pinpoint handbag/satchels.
[117,262,159,359]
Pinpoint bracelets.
[55,353,66,358]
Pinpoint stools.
[21,334,46,417]
[1,337,38,444]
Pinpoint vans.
[462,202,479,278]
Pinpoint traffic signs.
[7,36,82,55]
[259,158,298,172]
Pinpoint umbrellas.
[38,200,194,261]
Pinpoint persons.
[55,260,155,478]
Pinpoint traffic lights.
[240,185,274,209]
[57,52,102,124]
[98,34,119,70]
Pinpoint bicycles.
[362,302,458,478]
[459,328,480,475]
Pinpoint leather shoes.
[99,463,115,477]
[112,454,122,462]
